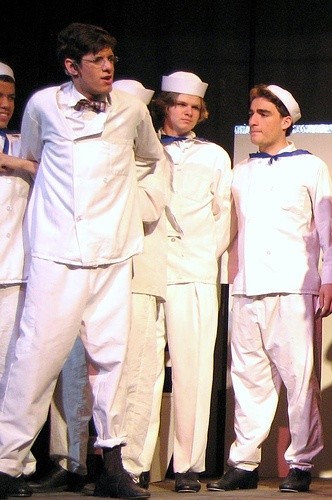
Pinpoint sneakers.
[0,471,32,500]
[207,467,259,491]
[26,463,88,492]
[279,468,312,492]
[174,469,202,492]
[93,465,151,498]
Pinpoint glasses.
[65,55,119,66]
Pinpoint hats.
[266,85,302,126]
[161,71,209,99]
[0,61,15,81]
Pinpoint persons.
[0,24,173,500]
[0,63,155,491]
[116,69,232,492]
[206,83,332,494]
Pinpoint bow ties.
[73,99,106,114]
